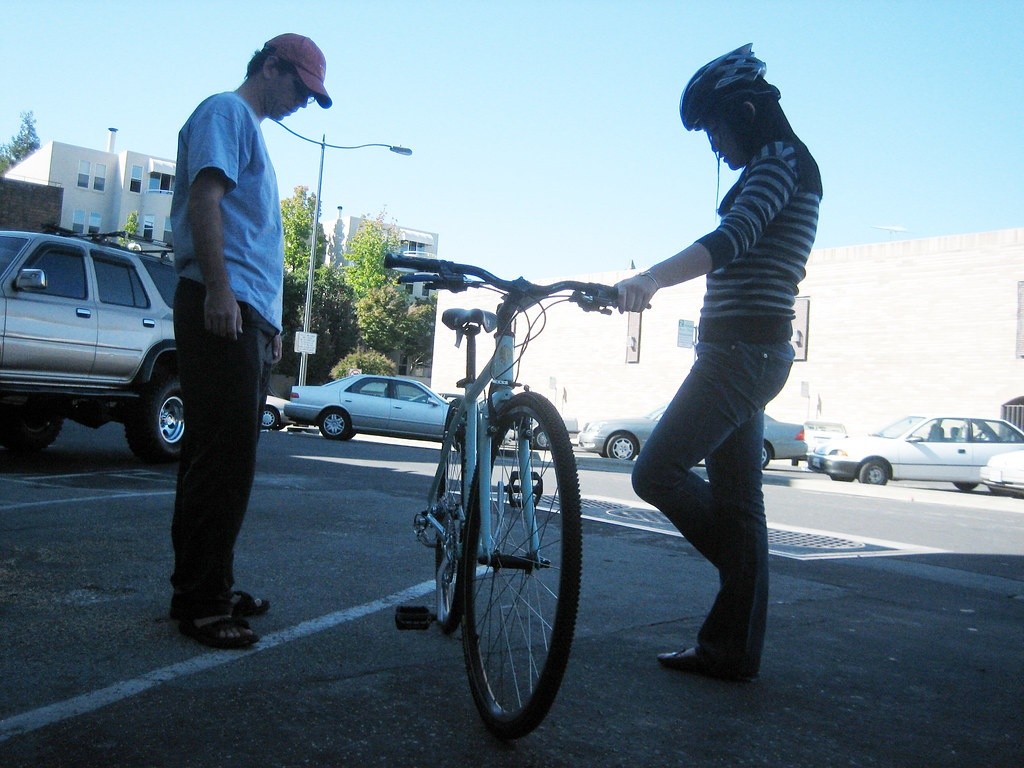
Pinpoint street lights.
[266,114,412,385]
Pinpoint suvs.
[0,226,192,463]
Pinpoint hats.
[262,33,332,108]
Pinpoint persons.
[169,32,332,648]
[612,43,823,686]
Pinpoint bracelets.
[639,273,659,290]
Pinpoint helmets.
[680,43,781,130]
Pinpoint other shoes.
[658,649,756,681]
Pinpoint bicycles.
[380,248,655,740]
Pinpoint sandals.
[223,590,269,616]
[178,617,260,648]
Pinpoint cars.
[978,449,1024,498]
[408,392,464,407]
[260,393,301,433]
[284,373,453,445]
[580,398,809,470]
[804,413,1024,491]
[498,407,585,454]
